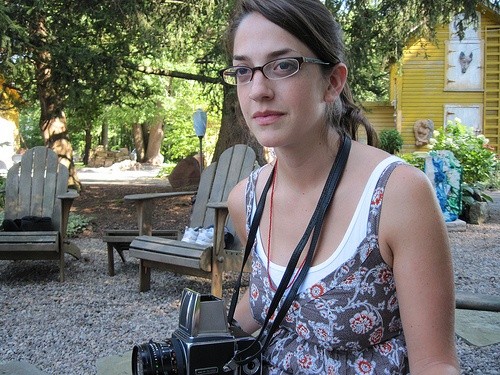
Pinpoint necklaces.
[267,159,307,294]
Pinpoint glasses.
[220,56,329,86]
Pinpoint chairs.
[124,144,263,300]
[0,146,80,282]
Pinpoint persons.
[217,0,463,374]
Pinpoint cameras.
[134,287,262,375]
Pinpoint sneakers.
[182,224,227,248]
